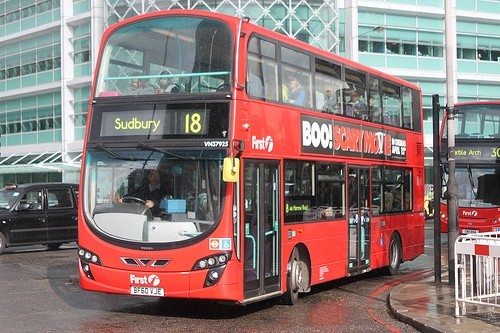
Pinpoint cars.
[0,182,79,255]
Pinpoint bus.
[77,9,425,307]
[437,100,500,238]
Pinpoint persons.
[153,71,178,93]
[324,88,337,111]
[427,186,435,217]
[266,78,288,101]
[127,72,153,94]
[392,174,410,211]
[370,96,380,122]
[119,170,168,218]
[346,90,367,120]
[287,75,305,105]
